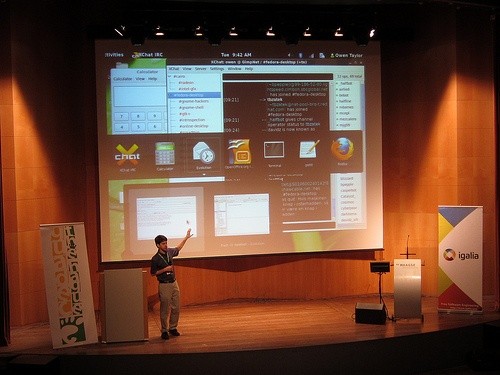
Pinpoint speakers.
[355,302,387,325]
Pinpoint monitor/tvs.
[264,141,285,159]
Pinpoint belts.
[159,278,177,284]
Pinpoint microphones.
[407,235,409,260]
[168,261,174,273]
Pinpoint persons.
[150,228,193,340]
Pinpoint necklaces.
[158,252,169,265]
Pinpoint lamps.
[208,33,222,47]
[130,34,144,47]
[356,35,369,46]
[286,34,299,45]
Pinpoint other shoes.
[169,328,180,336]
[161,331,169,339]
